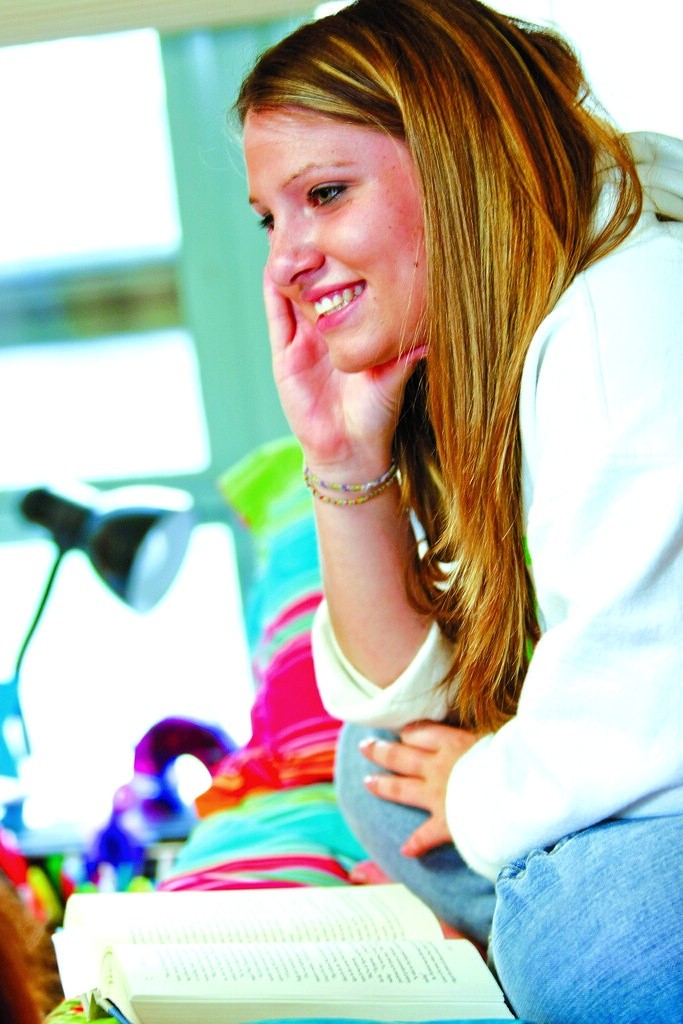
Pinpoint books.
[52,884,521,1024]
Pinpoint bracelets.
[304,462,397,506]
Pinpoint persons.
[229,0,683,1024]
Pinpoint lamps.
[7,485,190,687]
